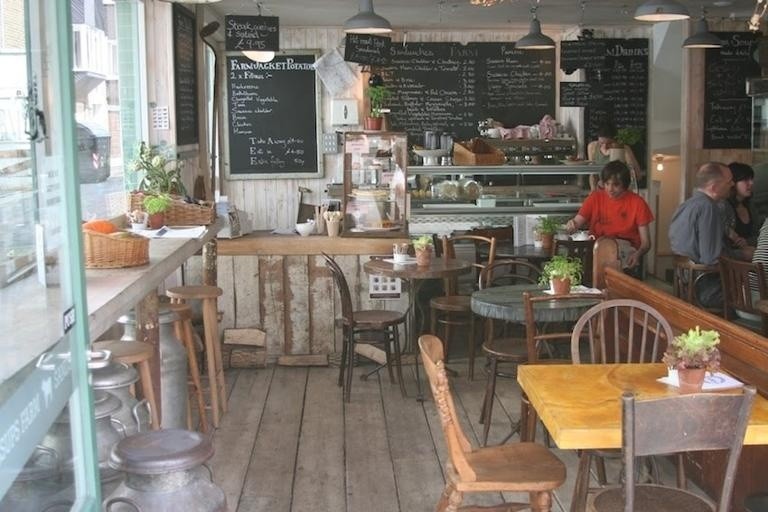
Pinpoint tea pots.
[569,232,597,242]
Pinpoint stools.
[90,285,228,431]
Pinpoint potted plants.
[143,194,170,228]
[535,215,563,248]
[609,125,642,164]
[363,85,391,130]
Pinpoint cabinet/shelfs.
[343,130,409,223]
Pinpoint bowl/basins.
[295,223,314,237]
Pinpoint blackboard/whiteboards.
[223,49,324,181]
[343,32,392,66]
[703,31,763,149]
[560,38,649,189]
[370,41,556,147]
[224,13,280,50]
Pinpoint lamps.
[342,0,394,36]
[634,0,724,49]
[513,0,555,50]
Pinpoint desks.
[0,212,224,405]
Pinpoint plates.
[412,149,450,157]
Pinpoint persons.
[562,158,654,283]
[585,126,643,199]
[667,161,747,311]
[717,158,768,264]
[733,210,768,323]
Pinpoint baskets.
[454,140,505,165]
[82,224,150,269]
[129,190,216,225]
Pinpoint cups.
[325,220,341,237]
[392,243,409,263]
